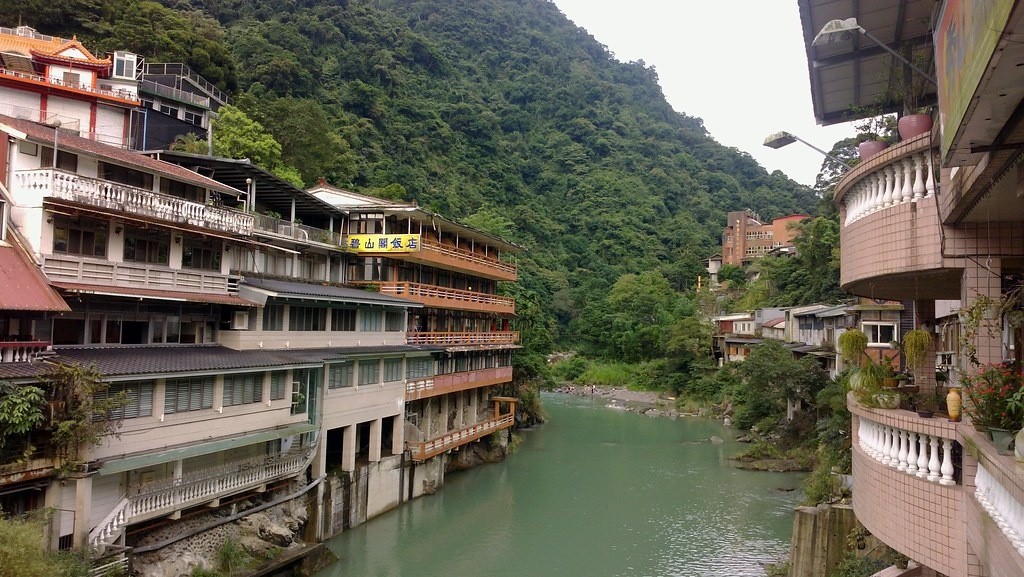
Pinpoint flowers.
[960,360,1024,430]
[914,399,933,412]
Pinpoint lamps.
[175,233,182,245]
[115,222,123,236]
[763,131,854,171]
[225,245,232,253]
[47,215,55,224]
[811,17,938,87]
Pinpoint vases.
[946,388,962,422]
[917,411,934,417]
[884,377,898,386]
[973,421,991,433]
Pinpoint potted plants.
[897,374,908,387]
[820,341,834,352]
[880,395,900,408]
[890,550,910,570]
[990,418,1019,456]
[876,42,932,141]
[841,103,889,161]
